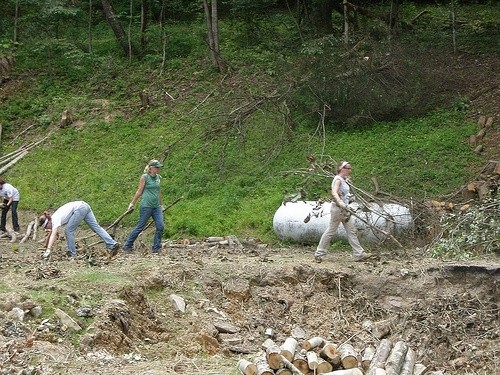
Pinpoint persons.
[123,159,166,253]
[0,179,20,236]
[38,200,121,259]
[313,159,372,263]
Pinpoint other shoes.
[315,256,322,263]
[110,243,119,257]
[123,246,135,253]
[152,248,162,254]
[362,252,372,260]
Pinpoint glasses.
[344,168,351,169]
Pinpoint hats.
[149,160,163,168]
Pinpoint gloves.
[0,203,9,210]
[43,248,52,258]
[128,203,134,210]
